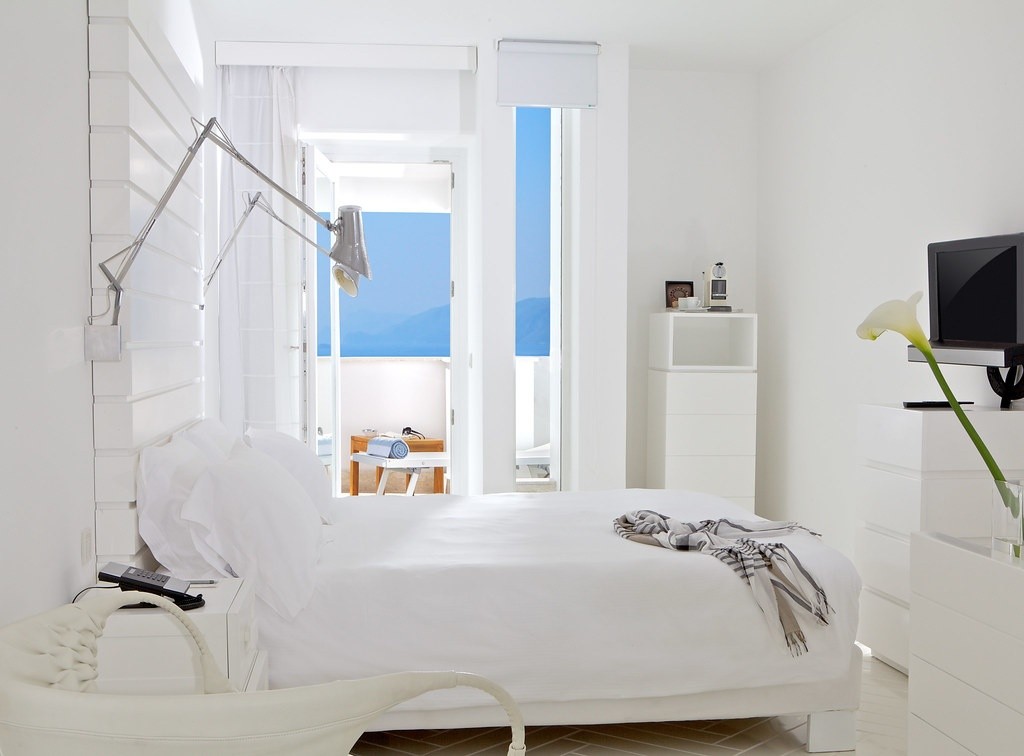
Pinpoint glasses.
[402,427,426,440]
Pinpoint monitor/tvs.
[929,231,1024,348]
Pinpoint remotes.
[903,402,952,409]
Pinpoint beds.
[154,488,863,753]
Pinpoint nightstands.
[76,576,270,695]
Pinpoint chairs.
[0,590,526,756]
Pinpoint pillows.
[137,414,332,621]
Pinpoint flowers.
[856,288,1019,516]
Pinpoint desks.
[351,436,444,493]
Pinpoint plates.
[686,307,711,313]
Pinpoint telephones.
[98,562,205,611]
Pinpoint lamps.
[203,191,360,299]
[98,117,373,325]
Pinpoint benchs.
[352,450,551,493]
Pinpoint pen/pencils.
[186,580,215,584]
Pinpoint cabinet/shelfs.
[647,311,758,514]
[907,527,1024,756]
[848,404,1024,676]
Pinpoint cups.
[678,298,688,311]
[687,297,701,309]
